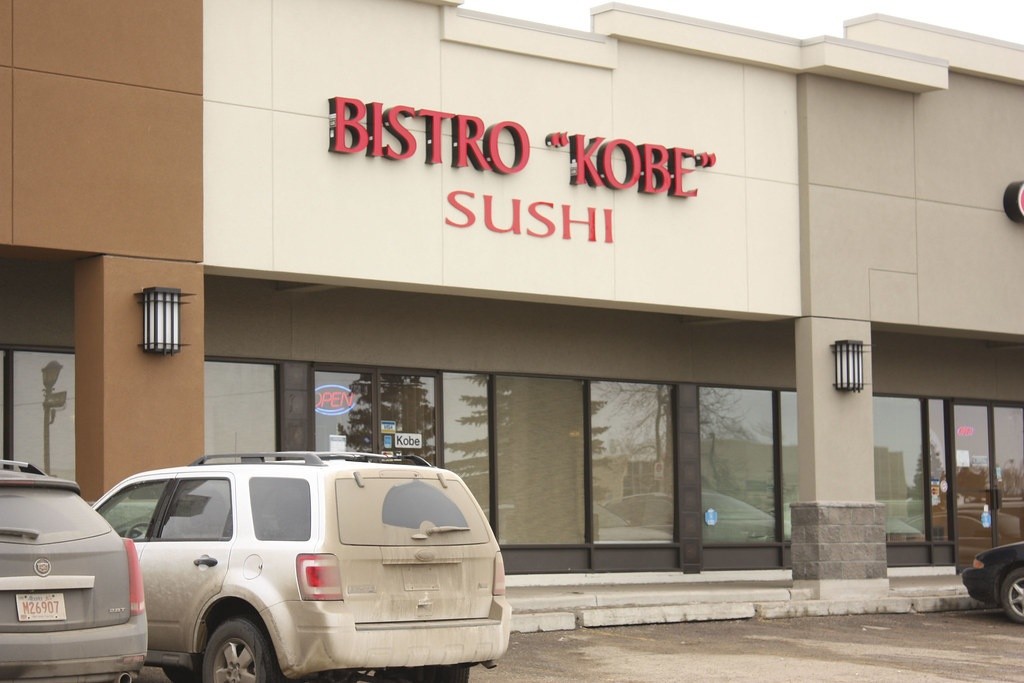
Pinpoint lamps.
[134,287,195,356]
[830,340,868,394]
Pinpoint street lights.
[40,359,63,476]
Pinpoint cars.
[961,540,1023,623]
[485,491,1024,564]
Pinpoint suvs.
[0,459,147,683]
[91,450,511,683]
